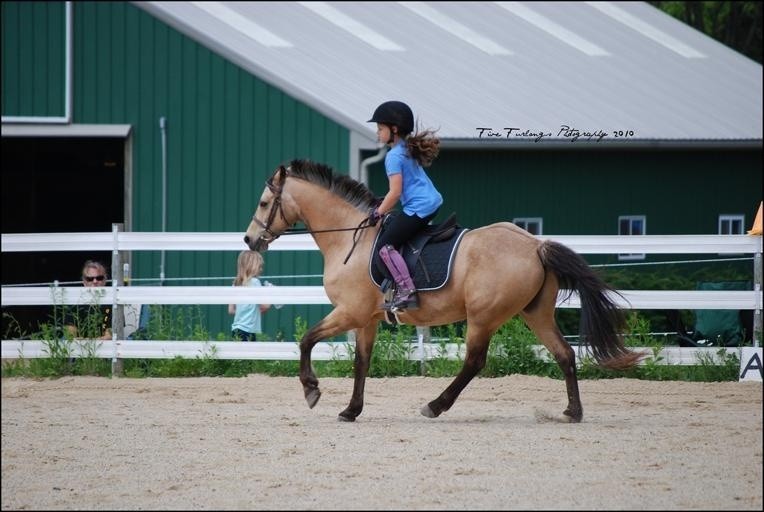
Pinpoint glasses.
[85,276,106,282]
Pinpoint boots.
[378,243,420,311]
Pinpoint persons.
[228,250,273,341]
[124,302,151,377]
[61,260,113,377]
[365,101,445,312]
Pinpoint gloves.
[369,208,384,227]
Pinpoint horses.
[245,159,650,424]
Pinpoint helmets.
[366,100,413,131]
